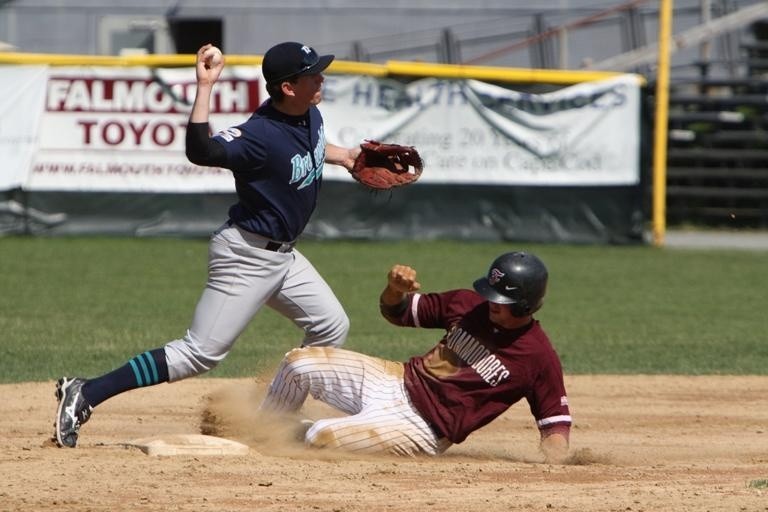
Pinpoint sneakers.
[52,376,93,449]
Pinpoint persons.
[260,249,574,467]
[43,38,426,451]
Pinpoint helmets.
[472,250,548,316]
[262,40,336,87]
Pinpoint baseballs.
[203,46,222,66]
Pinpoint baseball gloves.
[352,139,423,190]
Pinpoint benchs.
[642,16,766,221]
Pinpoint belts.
[264,240,295,254]
